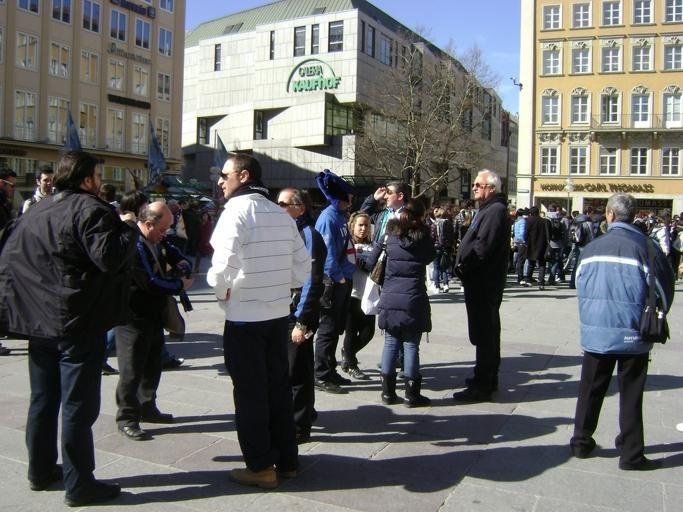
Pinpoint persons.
[111,200,197,441]
[276,186,327,447]
[206,154,311,486]
[414,164,682,291]
[565,192,673,470]
[314,168,436,409]
[1,166,231,376]
[2,150,141,508]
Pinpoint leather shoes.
[65,481,121,507]
[118,424,151,440]
[139,406,172,422]
[229,467,278,489]
[453,378,499,401]
[619,458,661,469]
[278,471,296,477]
[313,347,366,394]
[30,464,64,490]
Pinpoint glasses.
[275,201,305,208]
[472,183,491,188]
[2,180,15,188]
[219,171,240,178]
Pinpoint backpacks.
[575,221,588,247]
[319,277,336,312]
[434,219,455,249]
[514,218,527,244]
[648,228,660,243]
[547,216,563,242]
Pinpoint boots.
[380,373,397,404]
[191,256,201,273]
[404,374,430,407]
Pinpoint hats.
[317,169,359,210]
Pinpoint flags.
[64,104,81,153]
[148,114,167,190]
[214,128,228,171]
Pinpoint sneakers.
[101,364,118,374]
[520,276,564,289]
[569,285,576,288]
[0,343,11,355]
[433,284,464,295]
[161,358,184,371]
[377,359,404,377]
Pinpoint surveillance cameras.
[510,76,517,80]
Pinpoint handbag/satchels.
[163,295,185,334]
[371,249,387,285]
[440,249,452,274]
[544,240,562,264]
[362,276,381,315]
[640,297,669,344]
[176,214,187,239]
